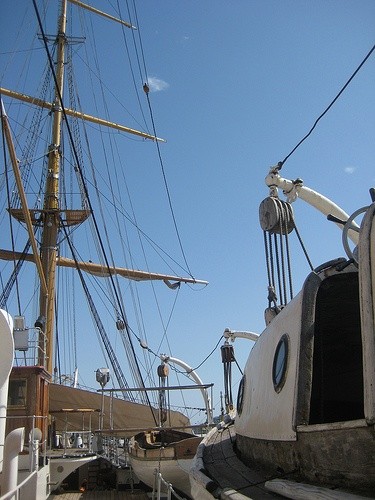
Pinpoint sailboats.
[0,1,211,500]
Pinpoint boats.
[190,162,374,500]
[128,329,263,496]
